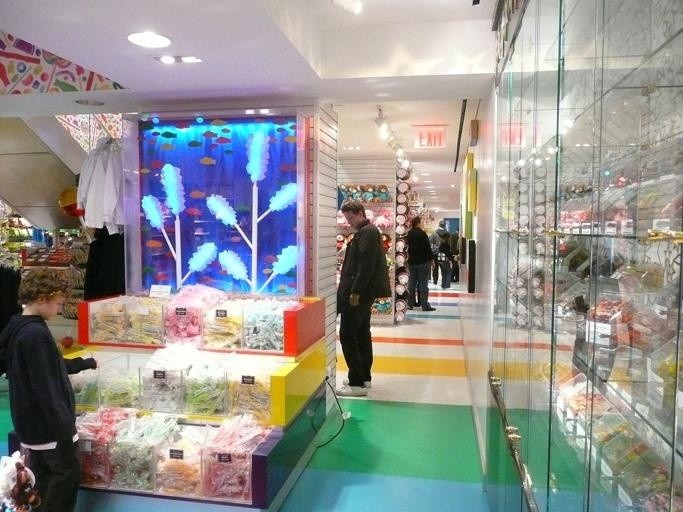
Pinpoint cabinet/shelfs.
[494,134,563,334]
[0,217,97,338]
[336,187,393,319]
[395,168,434,322]
[526,131,683,511]
[62,293,325,509]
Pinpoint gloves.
[81,358,96,369]
[57,430,73,458]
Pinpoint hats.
[439,221,445,228]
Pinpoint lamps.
[374,105,420,182]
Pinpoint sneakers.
[336,380,371,396]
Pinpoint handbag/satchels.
[437,252,447,262]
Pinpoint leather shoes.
[422,307,435,311]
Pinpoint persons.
[429,220,463,296]
[336,198,395,394]
[405,216,435,315]
[1,264,83,512]
